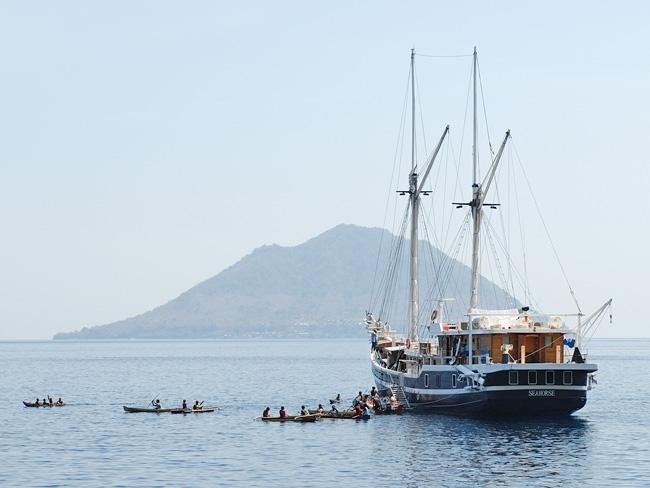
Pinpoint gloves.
[23,401,49,407]
[123,405,214,413]
[262,393,386,422]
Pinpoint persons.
[35,395,63,406]
[183,400,187,409]
[152,399,161,409]
[193,401,203,410]
[371,329,387,368]
[262,386,396,417]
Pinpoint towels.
[461,323,467,330]
[503,354,508,363]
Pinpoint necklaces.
[363,45,612,420]
